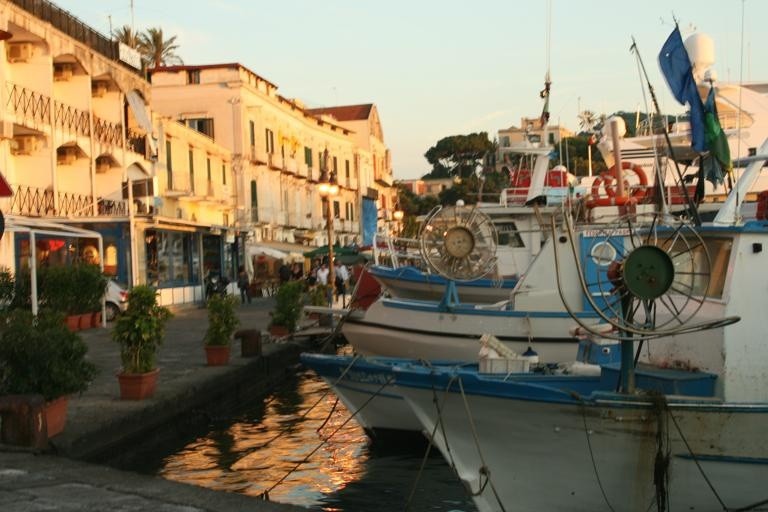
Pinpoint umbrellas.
[304,245,353,258]
[333,255,363,264]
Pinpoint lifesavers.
[605,162,648,205]
[591,170,630,199]
[587,197,628,211]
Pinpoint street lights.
[316,149,341,308]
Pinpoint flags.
[658,27,731,188]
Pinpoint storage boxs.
[479,357,529,374]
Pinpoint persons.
[278,257,348,301]
[237,269,251,304]
